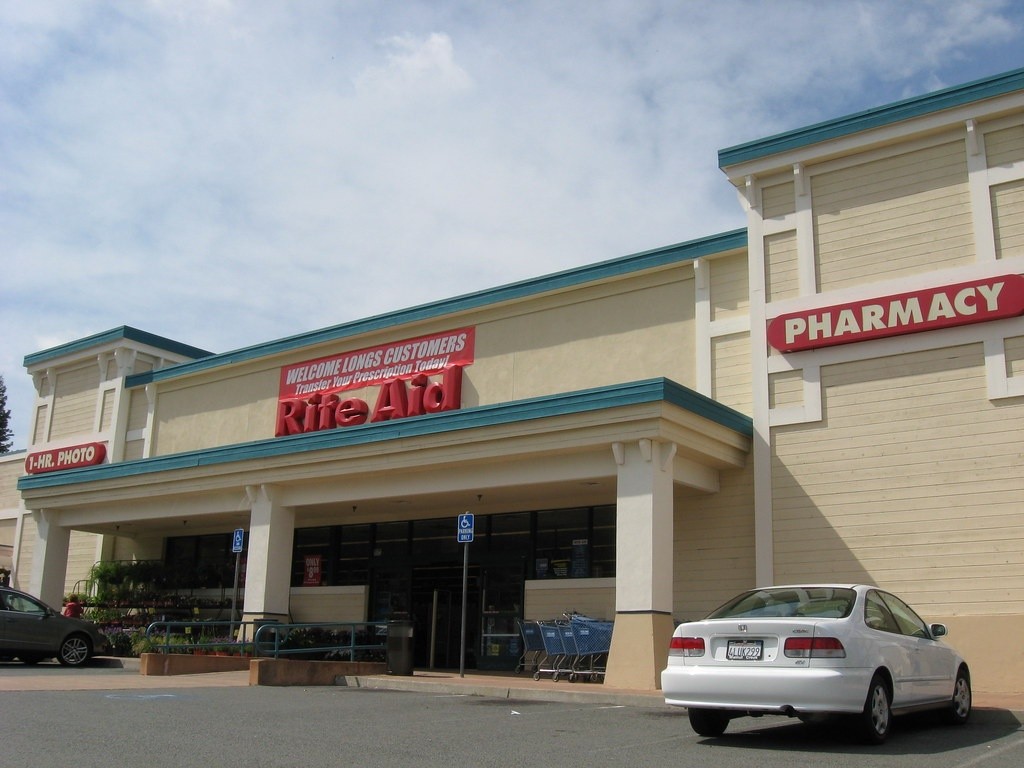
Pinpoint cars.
[0,587,106,668]
[660,582,973,746]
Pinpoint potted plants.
[97,611,109,623]
[122,614,135,625]
[119,591,132,605]
[105,590,118,605]
[135,613,146,624]
[110,611,121,624]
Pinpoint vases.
[165,599,176,607]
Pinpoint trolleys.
[515,610,614,683]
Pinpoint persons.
[63,593,84,620]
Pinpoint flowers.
[163,591,176,600]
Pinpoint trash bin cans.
[386,619,413,678]
